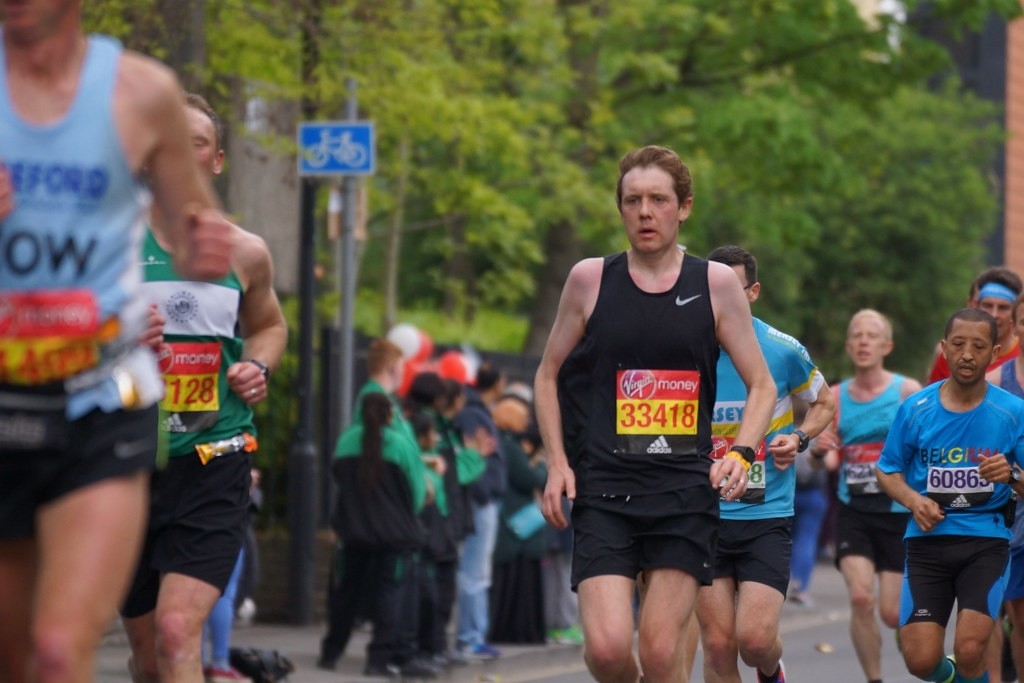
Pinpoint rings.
[251,389,257,396]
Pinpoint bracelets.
[811,444,823,460]
[248,359,269,379]
[726,452,750,472]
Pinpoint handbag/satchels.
[507,503,548,541]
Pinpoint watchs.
[731,446,755,464]
[794,430,808,453]
[1008,466,1021,484]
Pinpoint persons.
[532,147,777,683]
[789,267,1024,683]
[320,323,577,682]
[695,247,834,683]
[0,0,285,683]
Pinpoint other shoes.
[546,625,587,647]
[456,641,501,661]
[317,647,469,678]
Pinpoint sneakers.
[758,657,786,683]
